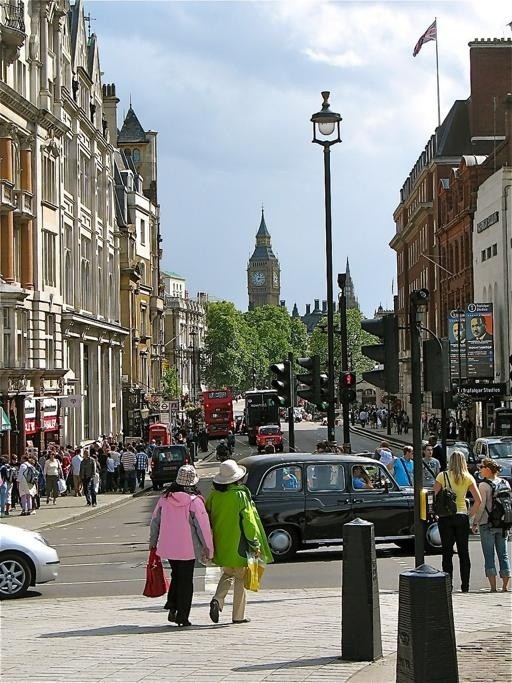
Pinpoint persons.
[1,409,249,518]
[351,464,374,489]
[386,444,414,485]
[472,457,511,593]
[374,443,392,460]
[433,450,482,593]
[374,440,394,473]
[303,401,475,445]
[148,463,215,626]
[470,314,492,340]
[263,438,351,490]
[421,444,441,487]
[205,459,261,623]
[423,435,446,471]
[184,392,188,401]
[451,319,466,344]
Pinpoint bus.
[200,389,235,440]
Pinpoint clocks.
[272,270,279,285]
[251,270,266,286]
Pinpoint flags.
[413,19,436,57]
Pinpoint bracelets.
[472,521,477,526]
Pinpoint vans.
[473,434,512,487]
[149,443,193,491]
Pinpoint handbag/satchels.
[238,510,258,559]
[57,478,67,493]
[142,549,169,597]
[243,551,266,593]
[435,489,458,517]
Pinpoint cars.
[234,401,325,436]
[422,437,489,487]
[0,521,61,600]
[254,422,286,452]
[238,450,444,559]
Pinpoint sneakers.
[168,611,177,622]
[0,505,40,518]
[103,488,136,494]
[232,617,251,624]
[210,600,219,623]
[46,498,56,506]
[92,501,98,507]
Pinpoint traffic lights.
[296,353,322,408]
[317,370,330,412]
[270,361,295,409]
[339,369,358,403]
[357,312,402,395]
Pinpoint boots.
[460,570,469,592]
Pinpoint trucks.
[244,388,283,446]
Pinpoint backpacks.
[487,479,512,532]
[26,465,39,485]
[5,465,20,484]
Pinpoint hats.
[175,464,201,486]
[211,458,248,485]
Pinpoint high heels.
[175,614,191,627]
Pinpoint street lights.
[309,89,345,452]
[189,327,199,402]
